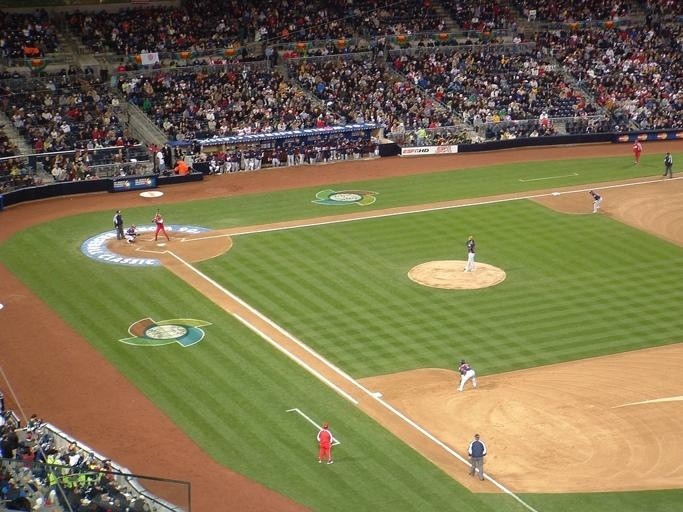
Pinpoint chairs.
[0,0,683,194]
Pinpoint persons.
[468,433,486,481]
[463,235,475,273]
[122,224,137,243]
[0,1,683,198]
[316,424,334,464]
[151,212,170,241]
[588,190,602,214]
[0,391,151,512]
[456,359,476,391]
[112,209,126,240]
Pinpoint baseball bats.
[155,208,159,223]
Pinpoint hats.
[473,434,480,438]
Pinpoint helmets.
[131,224,136,228]
[324,424,328,429]
[459,360,465,366]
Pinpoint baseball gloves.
[136,233,140,236]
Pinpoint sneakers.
[318,459,334,465]
